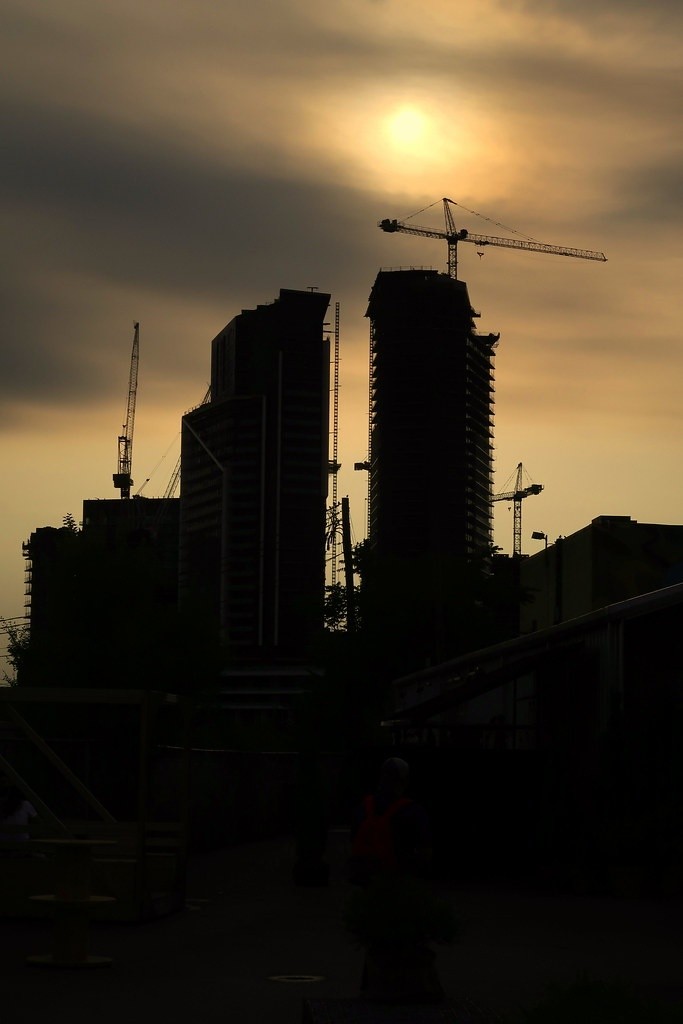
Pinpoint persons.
[0,783,39,842]
[352,743,433,998]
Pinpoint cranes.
[490,462,544,560]
[113,320,139,499]
[377,198,607,280]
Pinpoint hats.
[383,757,407,782]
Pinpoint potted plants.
[339,879,465,1003]
